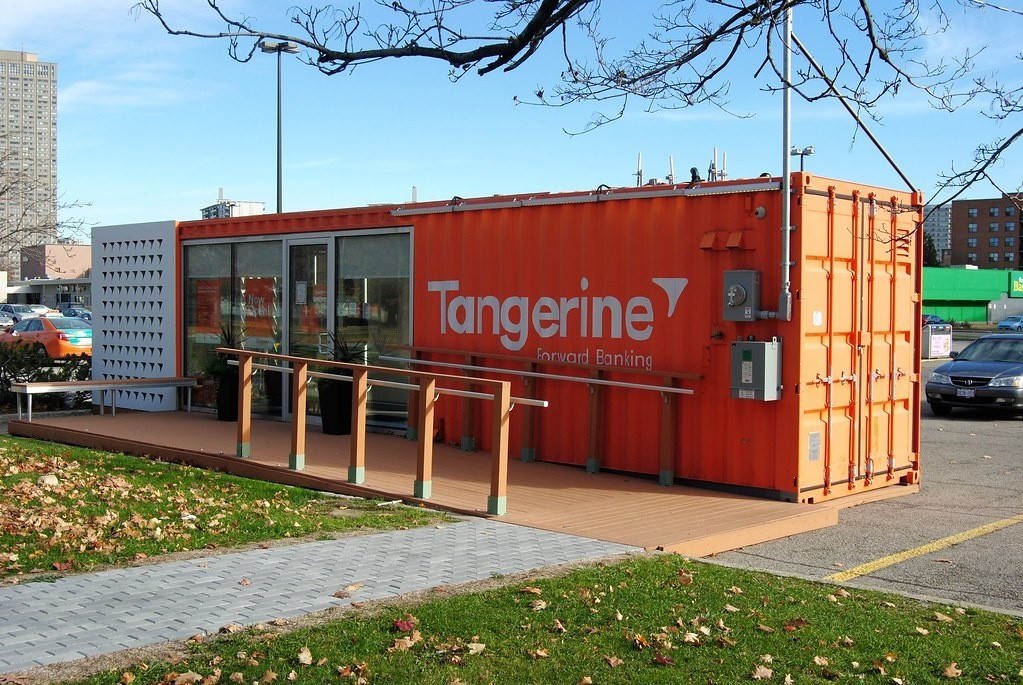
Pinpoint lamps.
[390,172,780,218]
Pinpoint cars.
[0,317,92,365]
[0,311,15,330]
[925,334,1023,416]
[52,302,85,313]
[26,305,49,315]
[998,316,1023,332]
[0,304,40,324]
[58,308,93,327]
[922,314,944,326]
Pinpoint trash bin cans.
[922,323,953,359]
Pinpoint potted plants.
[263,336,314,416]
[211,320,253,420]
[316,331,373,435]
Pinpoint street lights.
[258,41,300,214]
[791,146,815,172]
